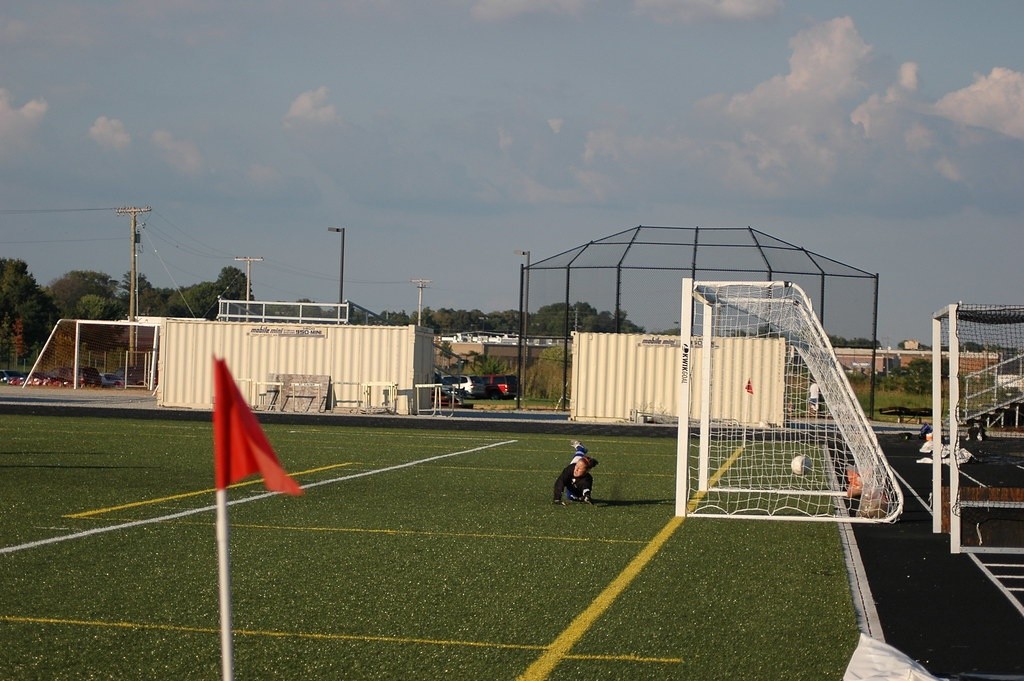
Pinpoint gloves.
[584,494,594,506]
[555,500,568,506]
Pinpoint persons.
[809,381,822,415]
[554,438,599,505]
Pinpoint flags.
[211,356,300,499]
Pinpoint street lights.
[512,249,530,404]
[327,227,344,317]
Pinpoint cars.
[99,367,157,387]
[0,369,51,383]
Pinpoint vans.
[52,366,102,387]
[439,374,518,401]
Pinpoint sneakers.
[570,439,588,453]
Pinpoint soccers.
[791,455,812,475]
